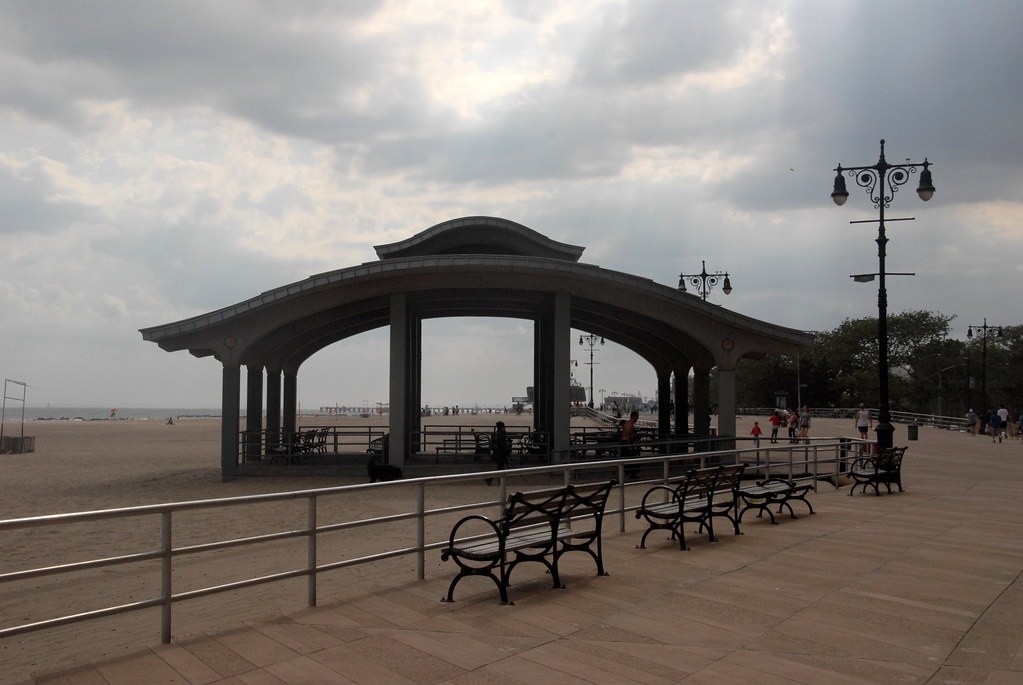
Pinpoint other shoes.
[993,440,995,442]
[630,474,639,479]
[485,479,491,485]
[1004,434,1008,439]
[770,441,778,444]
[789,441,794,444]
[999,437,1002,443]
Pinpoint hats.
[969,409,973,412]
[859,403,864,408]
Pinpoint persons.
[654,403,657,412]
[443,407,449,417]
[750,422,762,444]
[769,405,810,445]
[422,404,432,417]
[608,412,640,481]
[613,401,618,411]
[670,399,674,416]
[966,403,1023,442]
[854,403,872,439]
[650,401,653,414]
[485,421,508,485]
[451,405,459,416]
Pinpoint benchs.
[309,426,332,454]
[366,432,389,455]
[289,429,319,460]
[436,431,551,465]
[440,478,619,605]
[268,433,302,466]
[848,445,909,496]
[635,461,751,551]
[574,432,619,458]
[737,479,815,524]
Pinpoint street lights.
[829,138,936,481]
[967,319,1002,435]
[578,334,605,409]
[679,261,732,302]
